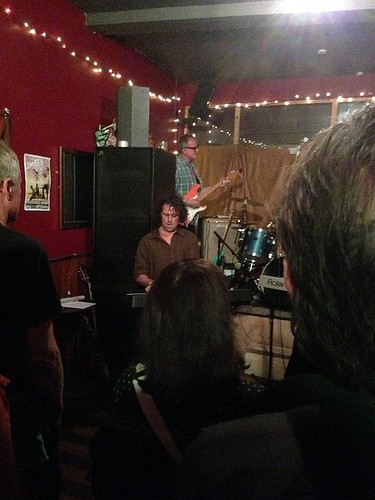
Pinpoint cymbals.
[228,197,265,206]
[235,210,264,223]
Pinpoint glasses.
[183,145,201,152]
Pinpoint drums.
[240,258,259,274]
[259,256,294,312]
[237,227,275,264]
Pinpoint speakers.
[198,217,243,267]
[91,146,176,293]
[189,80,216,117]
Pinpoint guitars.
[182,168,242,226]
[77,264,98,338]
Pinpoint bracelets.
[183,200,185,202]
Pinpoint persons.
[35,184,41,196]
[42,174,48,199]
[174,134,226,237]
[133,190,199,286]
[0,136,66,500]
[113,258,268,500]
[163,101,375,500]
[29,185,35,201]
